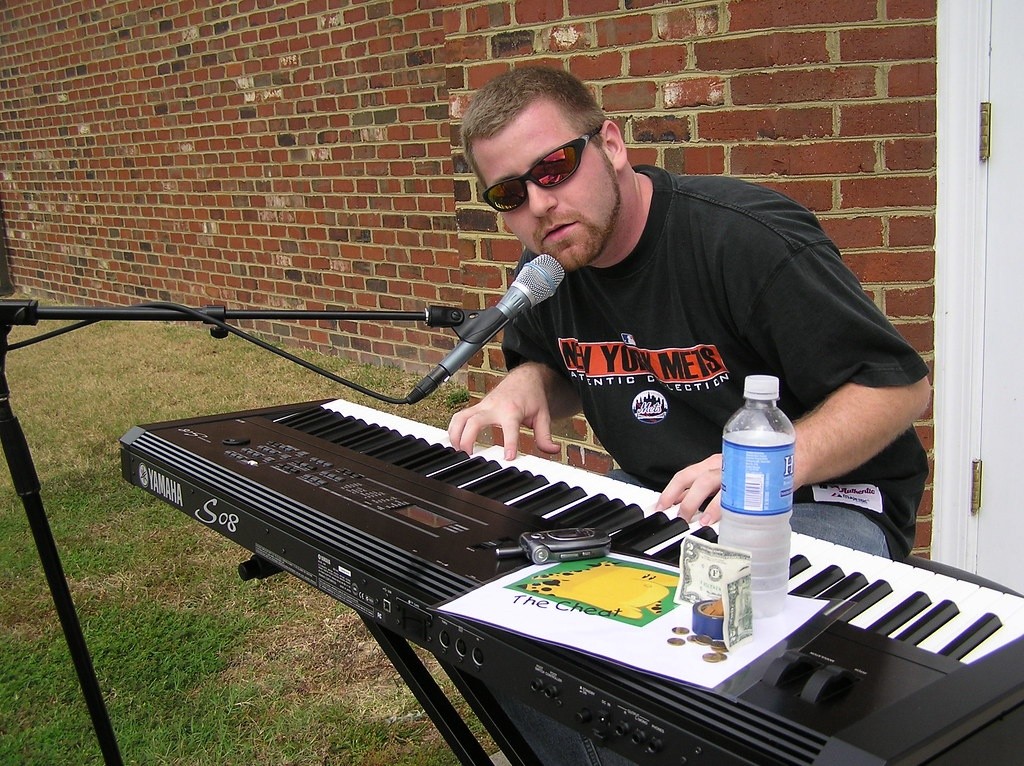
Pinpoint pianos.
[109,389,1023,766]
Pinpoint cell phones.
[496,528,612,565]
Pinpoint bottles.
[720,375,796,618]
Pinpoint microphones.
[407,253,565,405]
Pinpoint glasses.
[482,124,604,213]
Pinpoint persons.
[448,65,931,562]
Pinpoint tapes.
[692,597,723,641]
